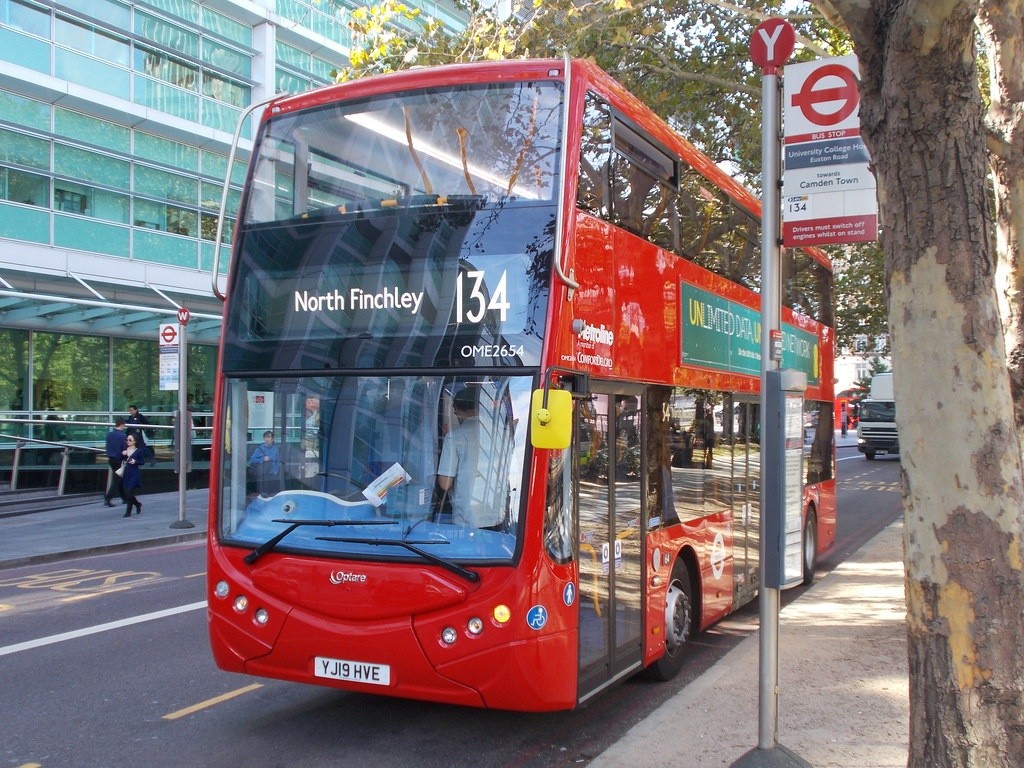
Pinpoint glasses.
[126,438,135,442]
[454,410,457,415]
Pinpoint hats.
[453,387,480,409]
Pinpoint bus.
[207,57,835,710]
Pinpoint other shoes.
[124,511,131,516]
[122,499,127,503]
[103,501,111,507]
[137,502,142,513]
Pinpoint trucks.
[856,374,901,459]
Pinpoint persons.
[104,405,155,518]
[250,431,282,478]
[437,387,515,535]
[703,411,715,468]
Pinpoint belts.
[479,523,503,532]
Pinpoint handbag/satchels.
[115,460,126,477]
[300,439,316,452]
[142,430,148,443]
[255,445,271,476]
